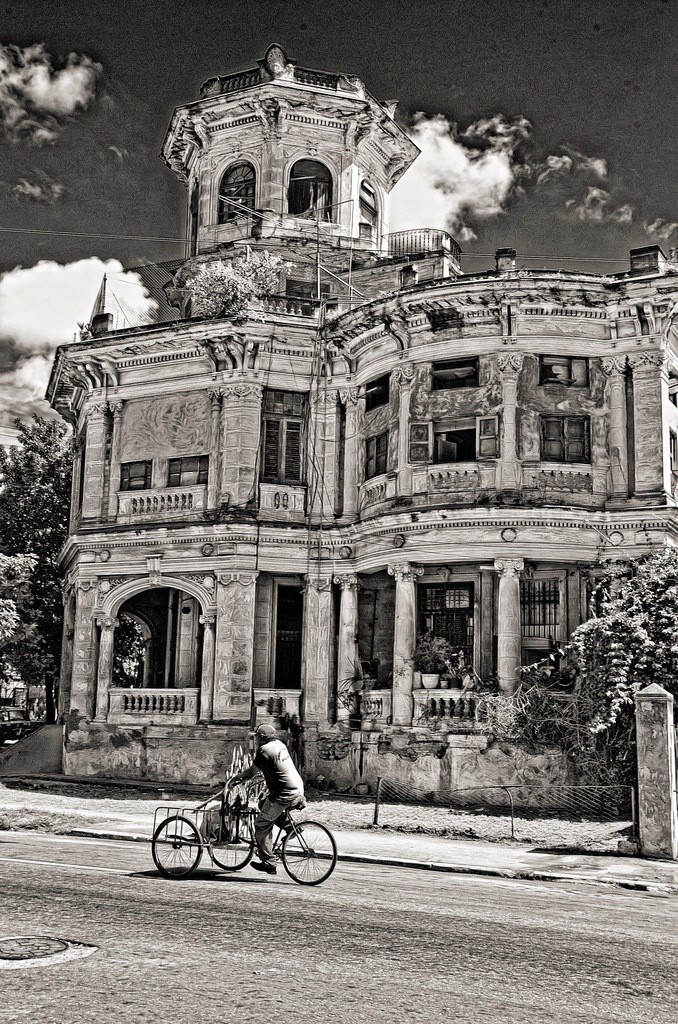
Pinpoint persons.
[229,725,304,872]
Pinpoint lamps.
[199,615,207,624]
[114,618,120,627]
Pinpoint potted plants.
[386,629,470,689]
[328,687,370,731]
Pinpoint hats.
[256,724,276,741]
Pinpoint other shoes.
[250,861,277,874]
[280,825,304,842]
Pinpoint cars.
[0,708,42,744]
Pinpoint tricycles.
[146,775,339,886]
